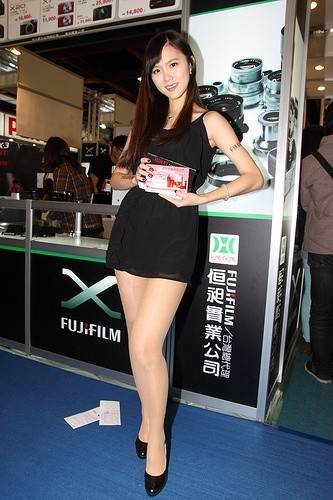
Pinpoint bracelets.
[220,184,229,201]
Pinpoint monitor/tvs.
[149,0,175,9]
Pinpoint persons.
[298,122,328,343]
[36,177,53,226]
[87,135,129,219]
[105,31,263,495]
[43,137,105,237]
[6,180,24,196]
[300,101,333,386]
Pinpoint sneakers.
[305,360,332,384]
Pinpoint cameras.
[58,15,73,28]
[93,4,112,21]
[20,20,37,35]
[57,1,74,15]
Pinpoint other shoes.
[145,437,168,496]
[136,435,148,459]
